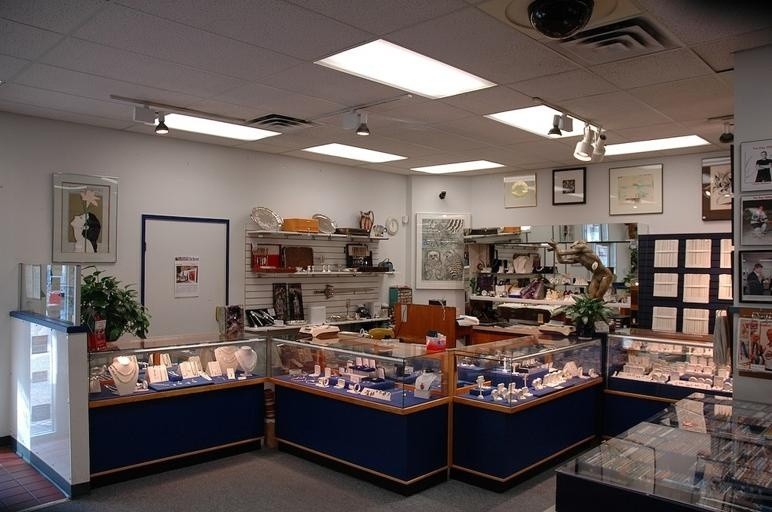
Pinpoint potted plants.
[81,265,150,350]
[551,292,615,341]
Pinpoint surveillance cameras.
[440,191,445,199]
[522,1,595,40]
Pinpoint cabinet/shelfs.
[470,240,631,346]
[245,230,394,331]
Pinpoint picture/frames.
[415,213,471,290]
[609,163,664,216]
[504,173,537,208]
[702,136,772,382]
[52,175,117,262]
[553,168,586,205]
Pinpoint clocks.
[385,217,399,236]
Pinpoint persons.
[275,295,287,315]
[548,238,614,302]
[744,205,770,238]
[71,210,103,253]
[292,296,301,314]
[746,262,770,296]
[739,330,766,366]
[762,328,772,371]
[753,150,771,183]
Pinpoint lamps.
[110,94,246,136]
[304,94,413,136]
[708,115,734,143]
[533,97,624,163]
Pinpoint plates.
[311,213,337,234]
[251,205,285,230]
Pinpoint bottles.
[358,210,375,232]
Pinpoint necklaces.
[90,345,257,393]
[416,374,436,390]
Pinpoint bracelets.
[323,377,329,387]
[353,383,361,393]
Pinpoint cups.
[372,224,390,237]
